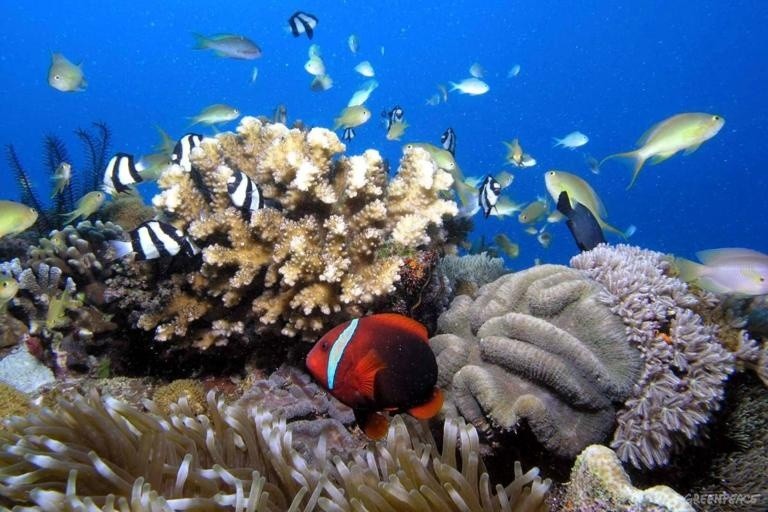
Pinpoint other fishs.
[101,11,637,307]
